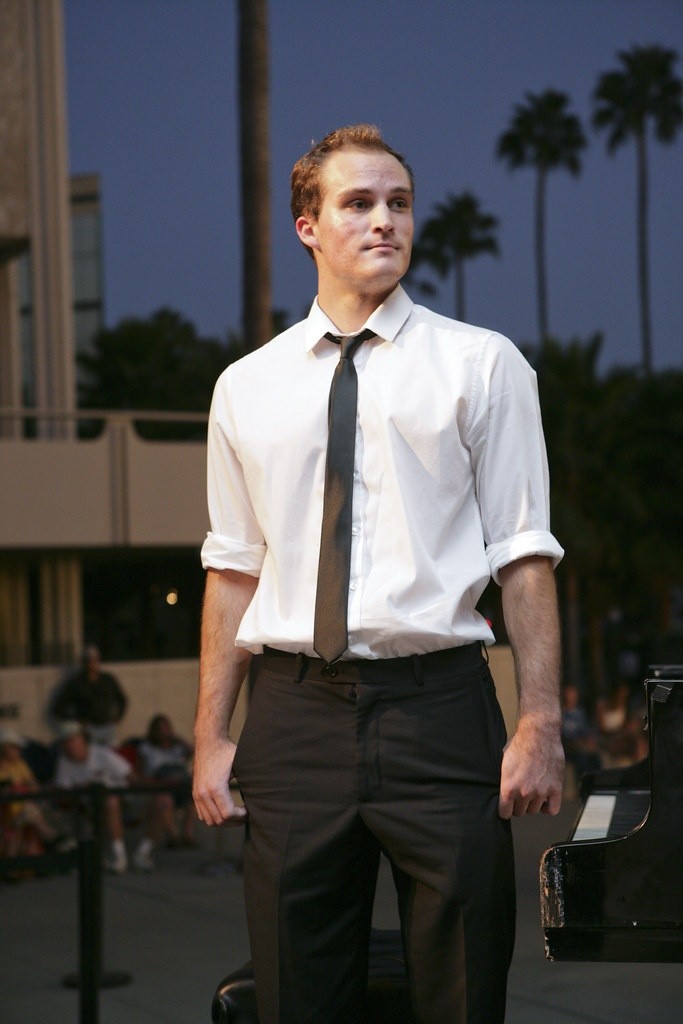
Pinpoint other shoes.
[108,849,128,874]
[131,851,154,871]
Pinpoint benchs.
[211,928,410,1024]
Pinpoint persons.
[0,645,645,883]
[194,123,567,1024]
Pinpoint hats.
[0,727,27,746]
[57,720,81,740]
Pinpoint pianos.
[532,660,683,965]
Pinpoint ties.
[312,329,381,659]
